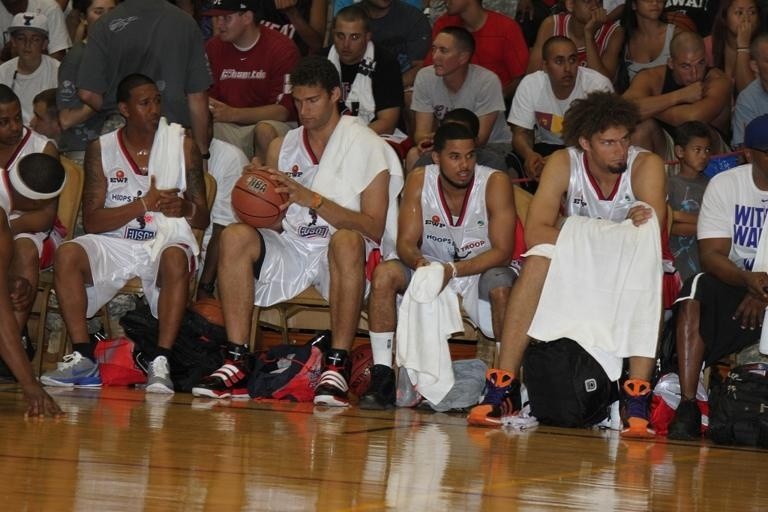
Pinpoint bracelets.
[309,191,323,210]
[447,261,457,279]
[140,198,148,211]
[185,201,197,221]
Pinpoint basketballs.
[232,169,291,228]
[189,298,225,341]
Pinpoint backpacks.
[119,304,227,393]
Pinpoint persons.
[35,71,209,395]
[190,59,390,408]
[466,90,663,440]
[0,152,67,421]
[2,0,766,371]
[666,114,767,442]
[357,121,516,411]
[0,82,66,344]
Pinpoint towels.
[525,198,665,381]
[395,261,469,406]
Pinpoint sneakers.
[314,348,349,407]
[618,378,656,439]
[145,355,176,394]
[191,341,249,399]
[359,364,396,410]
[467,369,520,426]
[667,393,700,441]
[39,351,103,387]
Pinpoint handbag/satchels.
[522,338,618,430]
[650,372,710,436]
[247,344,322,403]
[93,337,147,387]
[707,364,767,447]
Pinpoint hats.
[7,12,48,36]
[744,113,767,147]
[201,1,248,15]
